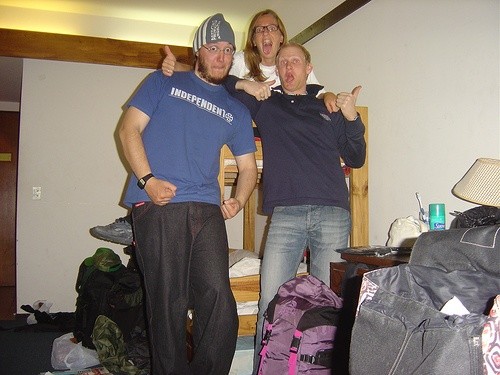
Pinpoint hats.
[192,13,237,56]
[84,249,123,272]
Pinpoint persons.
[89,8,341,246]
[222,43,367,375]
[118,14,259,375]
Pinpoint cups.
[419,211,430,232]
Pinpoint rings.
[167,69,170,73]
[261,95,265,98]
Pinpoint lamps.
[451,158,500,211]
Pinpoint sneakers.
[88,217,134,246]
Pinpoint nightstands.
[327,246,410,304]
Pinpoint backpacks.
[73,249,127,351]
[256,273,344,375]
[90,269,152,375]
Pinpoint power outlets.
[33,186,41,199]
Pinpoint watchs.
[354,115,360,121]
[137,173,155,190]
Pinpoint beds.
[220,105,369,336]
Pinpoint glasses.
[251,24,279,34]
[200,44,235,54]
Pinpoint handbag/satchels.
[27,299,55,325]
[348,226,500,375]
[50,332,101,371]
